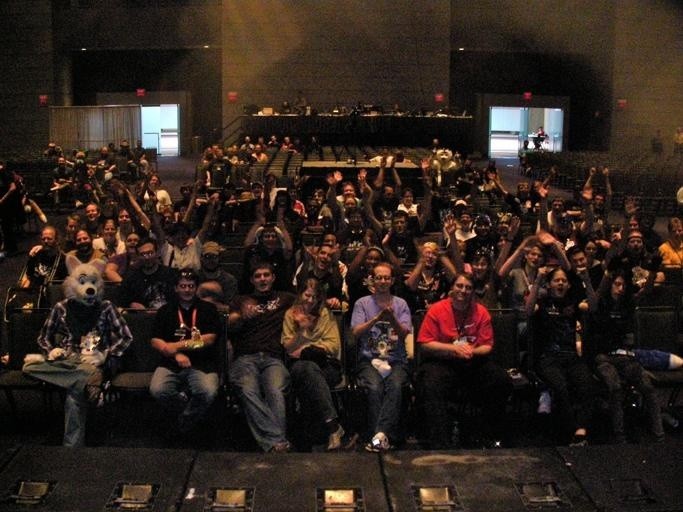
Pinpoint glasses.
[205,254,218,260]
[373,275,393,281]
[139,252,154,257]
[179,283,195,288]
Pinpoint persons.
[533,127,544,149]
[648,128,663,154]
[2,136,683,454]
[671,127,683,153]
[278,96,458,121]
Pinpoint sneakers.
[83,372,111,405]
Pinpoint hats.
[176,267,199,281]
[201,241,220,256]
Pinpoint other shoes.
[566,425,665,447]
[273,436,291,451]
[324,424,505,451]
